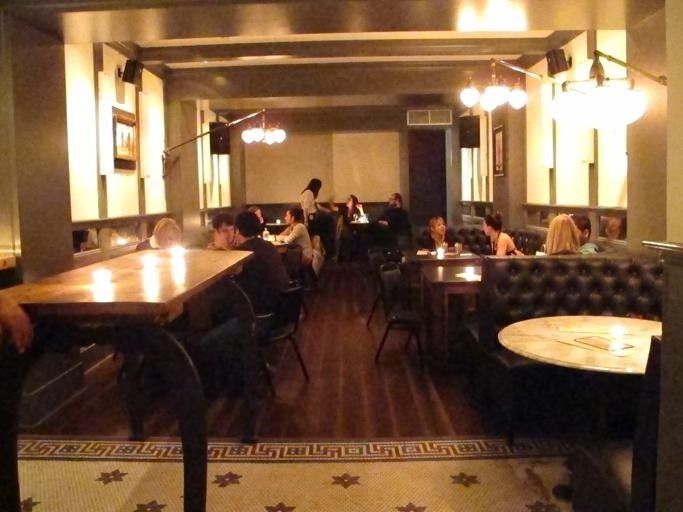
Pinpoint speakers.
[545,48,568,76]
[458,115,480,149]
[122,59,145,84]
[209,121,230,155]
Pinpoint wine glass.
[353,213,358,222]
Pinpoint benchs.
[0,218,662,512]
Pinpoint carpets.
[12,436,577,512]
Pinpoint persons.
[1,293,35,355]
[376,193,408,233]
[331,194,366,260]
[250,205,311,266]
[420,215,466,343]
[301,179,330,228]
[138,212,289,389]
[482,212,518,257]
[569,212,600,255]
[599,216,625,239]
[510,212,582,257]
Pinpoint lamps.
[549,48,667,132]
[164,109,287,149]
[458,56,549,111]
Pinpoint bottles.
[262,228,270,241]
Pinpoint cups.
[454,242,462,257]
